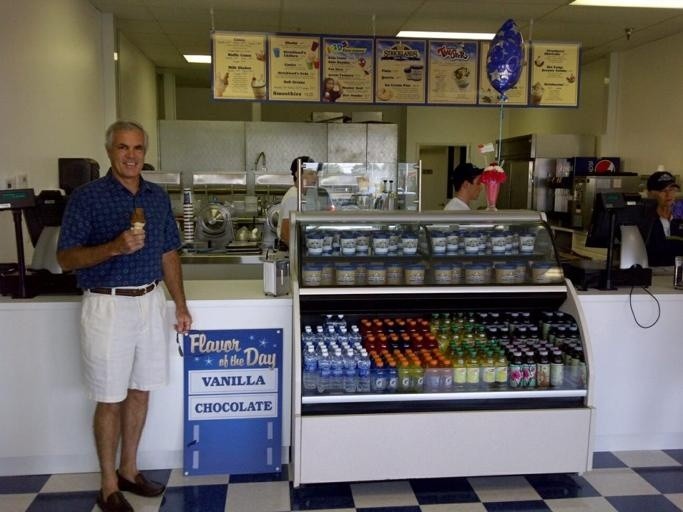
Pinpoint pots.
[350,195,369,208]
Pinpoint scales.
[0,188,83,299]
[578,191,652,291]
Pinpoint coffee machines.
[537,156,619,221]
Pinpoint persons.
[277,156,332,248]
[56,120,191,510]
[323,77,343,102]
[643,171,683,265]
[442,162,484,210]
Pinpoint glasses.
[177,330,206,356]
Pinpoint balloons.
[486,17,526,100]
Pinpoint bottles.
[302,312,585,391]
[382,180,395,210]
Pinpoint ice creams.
[130,208,146,230]
[480,162,506,206]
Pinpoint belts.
[91,280,160,296]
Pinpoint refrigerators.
[494,133,599,211]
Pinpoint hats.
[453,162,483,181]
[647,172,680,192]
[291,157,323,175]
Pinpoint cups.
[305,229,537,255]
[250,80,266,99]
[4,172,28,191]
[403,64,424,82]
[272,48,280,58]
[182,187,194,245]
[484,182,499,212]
[305,41,319,70]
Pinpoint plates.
[375,89,394,100]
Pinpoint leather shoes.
[96,488,134,512]
[116,469,165,497]
